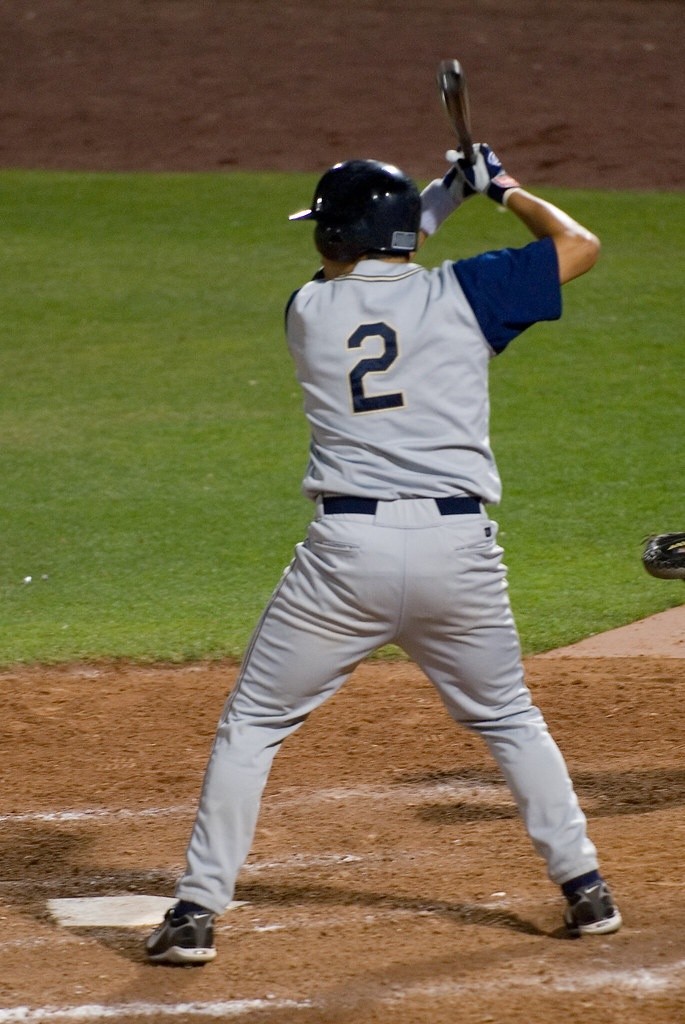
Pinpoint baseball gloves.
[640,530,685,580]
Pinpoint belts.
[322,496,481,516]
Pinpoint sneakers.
[562,879,623,935]
[145,911,218,963]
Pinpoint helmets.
[288,158,422,262]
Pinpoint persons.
[144,142,622,962]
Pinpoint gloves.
[446,142,522,206]
[441,165,478,205]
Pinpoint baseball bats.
[435,56,481,164]
